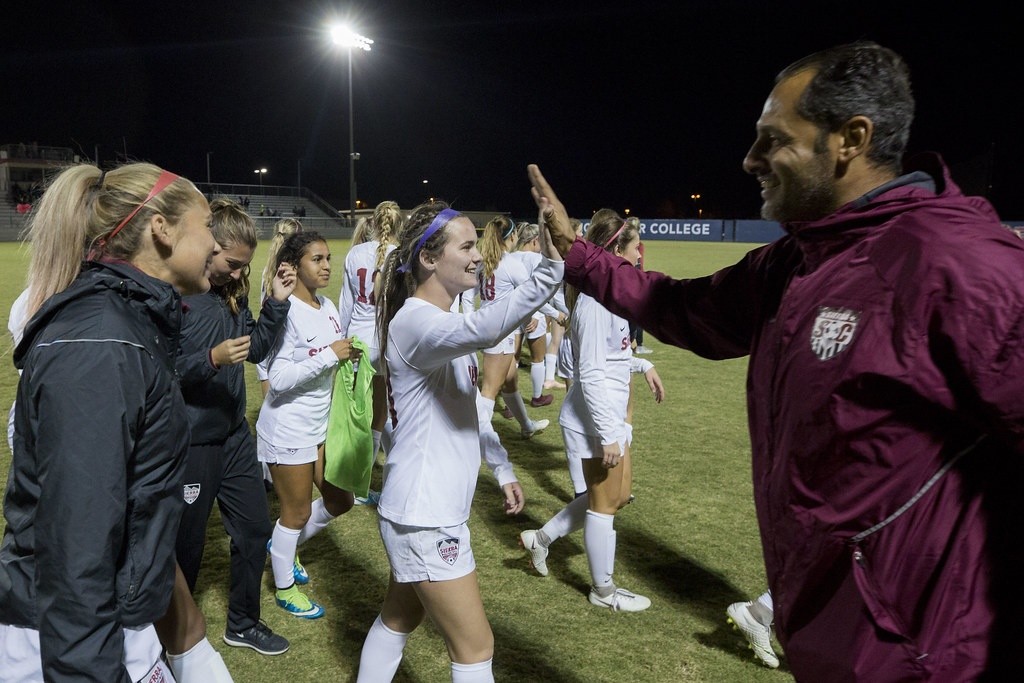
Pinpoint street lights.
[332,21,373,226]
[423,180,428,200]
[255,169,267,189]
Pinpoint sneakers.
[224,618,290,655]
[635,346,653,355]
[545,381,566,388]
[532,394,553,406]
[516,529,549,576]
[726,601,779,669]
[353,489,382,506]
[266,539,309,584]
[276,584,324,618]
[588,587,651,612]
[503,406,514,420]
[522,419,549,437]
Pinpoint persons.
[557,209,635,501]
[256,217,304,397]
[11,181,44,212]
[338,201,404,466]
[474,216,583,439]
[521,218,665,612]
[356,204,565,683]
[7,288,234,683]
[208,187,214,202]
[237,196,250,211]
[528,43,1024,683]
[267,207,282,221]
[174,196,290,656]
[727,588,779,669]
[0,162,221,683]
[292,205,306,223]
[627,216,653,353]
[256,232,364,619]
[18,141,38,158]
